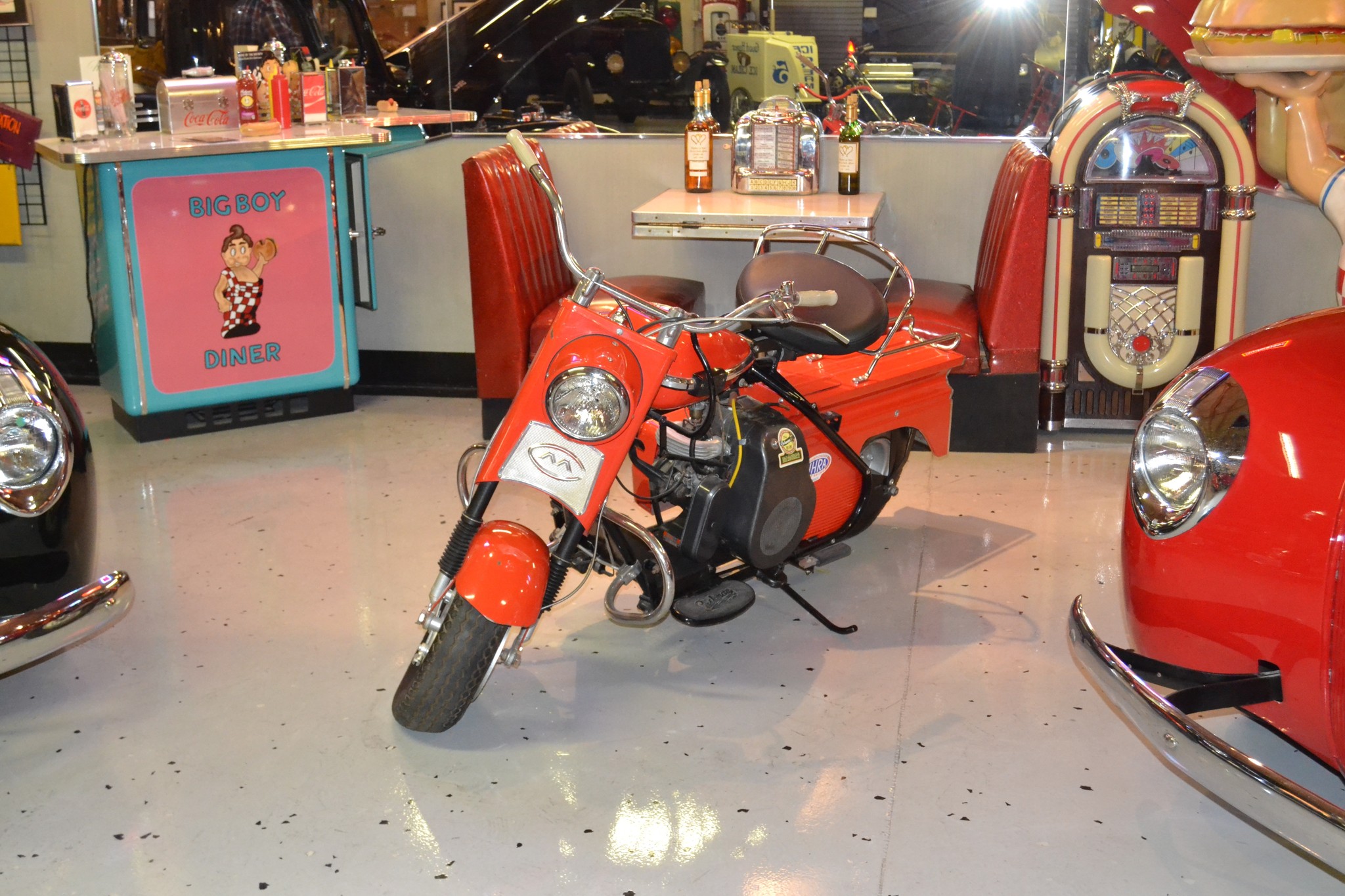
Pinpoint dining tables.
[632,189,889,256]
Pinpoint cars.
[524,12,691,125]
[1066,301,1344,887]
[0,314,134,676]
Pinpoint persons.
[1234,71,1345,307]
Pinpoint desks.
[333,105,479,140]
[33,118,392,443]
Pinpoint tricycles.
[726,27,885,137]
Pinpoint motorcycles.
[391,130,968,739]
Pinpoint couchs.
[865,140,1053,453]
[459,135,709,444]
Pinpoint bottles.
[838,96,861,194]
[702,79,720,134]
[686,81,714,194]
[851,94,863,135]
[236,64,293,129]
[327,57,341,116]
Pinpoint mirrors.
[89,0,1194,143]
